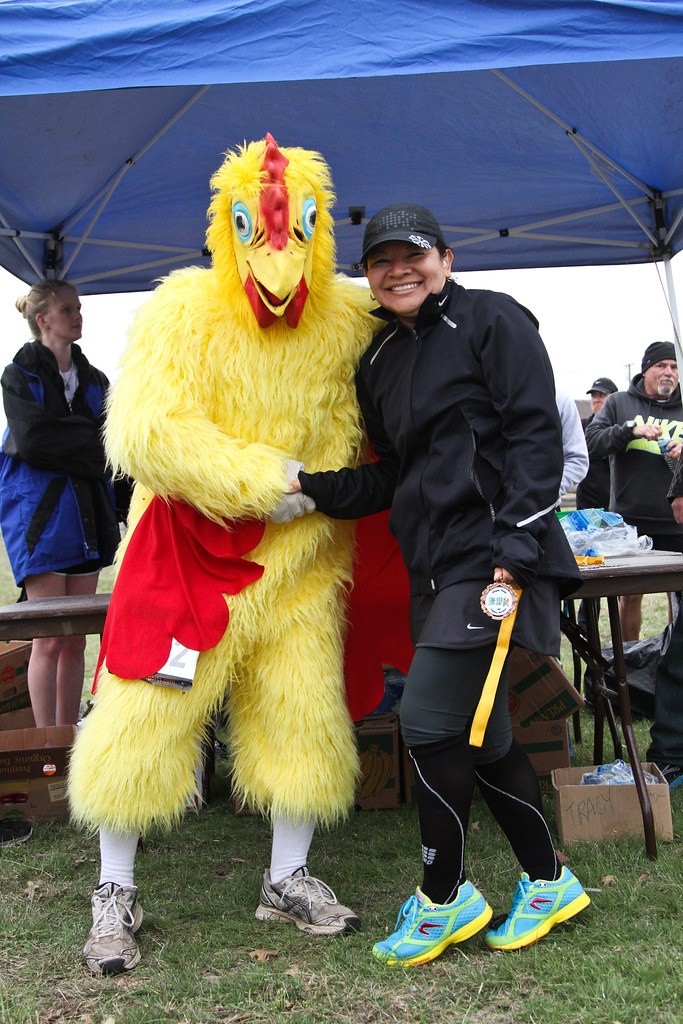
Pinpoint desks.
[560,549,683,861]
[0,591,114,640]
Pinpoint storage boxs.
[550,762,675,849]
[505,640,583,723]
[402,744,483,805]
[0,725,79,824]
[510,717,571,779]
[184,737,202,813]
[233,792,270,814]
[353,711,399,810]
[0,639,37,731]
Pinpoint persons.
[0,280,135,727]
[283,206,590,965]
[580,342,683,790]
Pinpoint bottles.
[658,432,679,474]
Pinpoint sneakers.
[371,880,493,968]
[647,761,683,792]
[485,866,591,949]
[83,882,144,975]
[255,866,361,935]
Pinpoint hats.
[641,342,676,374]
[586,378,619,395]
[359,204,444,264]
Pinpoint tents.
[0,0,683,406]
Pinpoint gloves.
[268,459,316,522]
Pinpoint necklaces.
[59,363,72,390]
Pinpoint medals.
[480,581,518,620]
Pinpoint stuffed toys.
[67,133,388,978]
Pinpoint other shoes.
[0,818,33,847]
